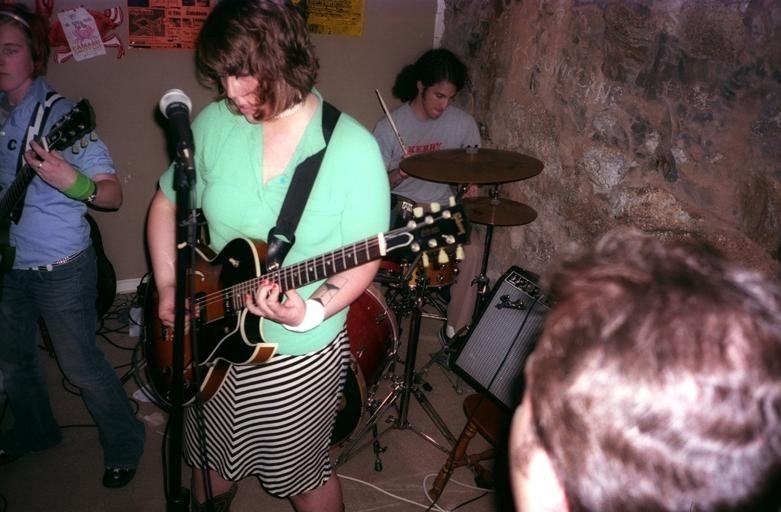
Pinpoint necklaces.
[274,99,303,119]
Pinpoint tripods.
[332,277,486,480]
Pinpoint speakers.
[449,266,559,414]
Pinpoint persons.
[0,3,145,488]
[507,225,780,511]
[371,49,480,354]
[146,0,390,512]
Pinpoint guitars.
[141,198,471,407]
[0,98,98,277]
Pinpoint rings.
[37,163,43,168]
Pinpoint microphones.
[160,87,201,187]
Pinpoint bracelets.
[282,299,325,334]
[61,172,99,206]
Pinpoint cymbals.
[458,196,540,227]
[399,148,543,184]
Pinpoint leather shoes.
[0,447,11,464]
[103,466,136,489]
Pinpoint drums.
[372,193,418,286]
[400,203,457,289]
[327,284,399,451]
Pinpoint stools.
[428,394,508,503]
